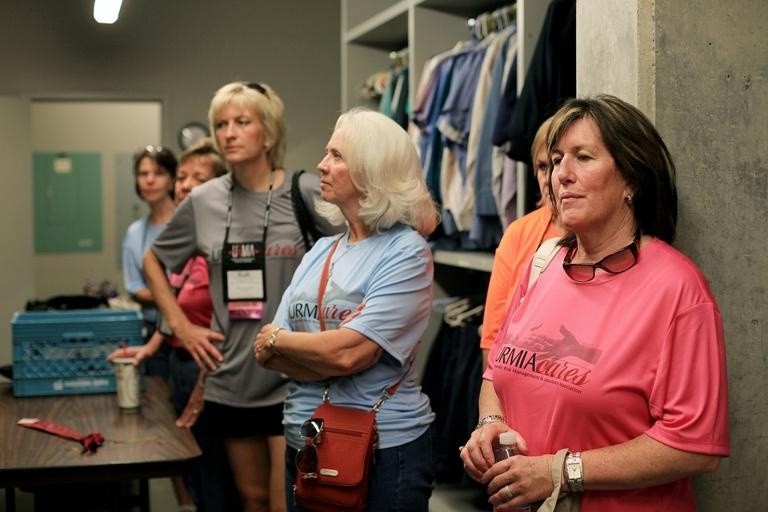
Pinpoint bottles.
[486,428,531,512]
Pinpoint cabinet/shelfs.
[340,1,561,511]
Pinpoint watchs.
[565,450,585,493]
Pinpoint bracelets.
[270,328,283,356]
[475,415,506,427]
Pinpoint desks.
[0,371,202,512]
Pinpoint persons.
[141,78,363,512]
[121,145,208,512]
[460,95,732,512]
[252,108,442,512]
[482,113,576,376]
[112,140,239,512]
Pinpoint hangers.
[466,2,516,39]
[379,47,411,70]
[430,296,485,330]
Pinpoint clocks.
[177,120,208,151]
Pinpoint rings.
[502,484,513,499]
[192,408,201,415]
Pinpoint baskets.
[11,309,143,398]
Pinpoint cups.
[109,355,145,414]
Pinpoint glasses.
[248,82,270,102]
[296,418,323,475]
[562,223,641,282]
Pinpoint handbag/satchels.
[294,401,376,512]
